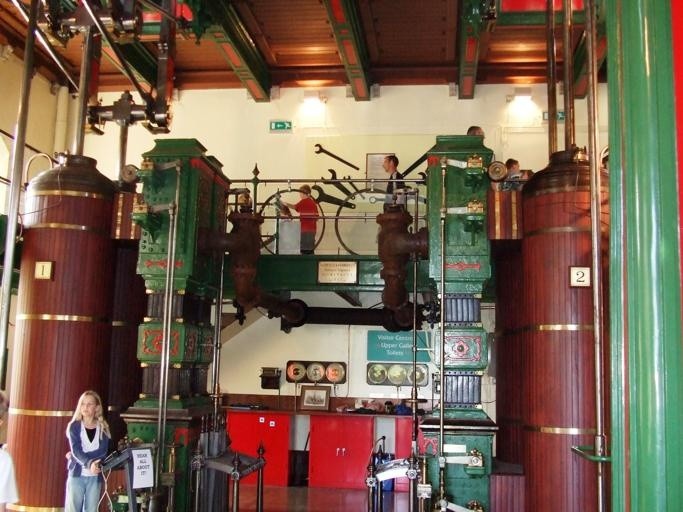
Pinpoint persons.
[467,126,484,136]
[63,390,111,512]
[381,156,407,211]
[0,390,20,512]
[283,184,320,254]
[498,158,529,191]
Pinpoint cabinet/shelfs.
[308,415,376,491]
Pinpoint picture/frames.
[299,385,332,412]
